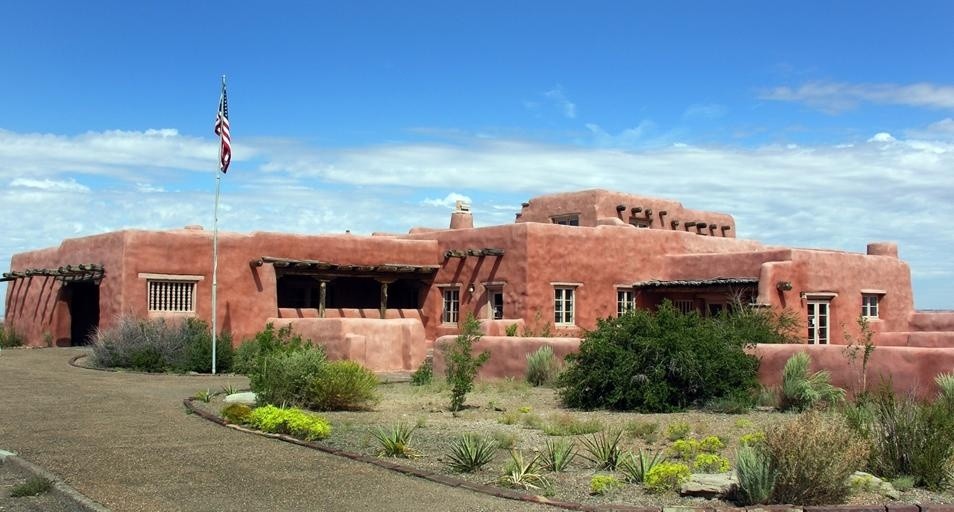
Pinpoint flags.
[214,87,232,174]
